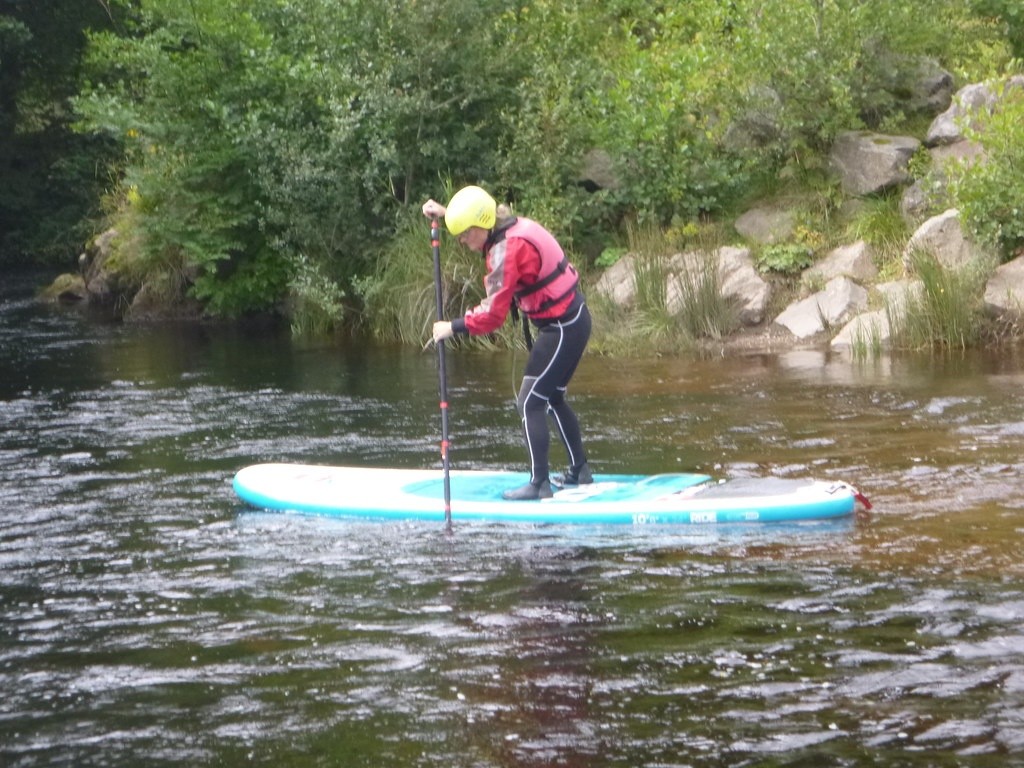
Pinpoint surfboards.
[229,460,857,525]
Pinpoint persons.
[422,185,594,500]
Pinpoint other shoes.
[502,479,553,500]
[551,461,594,484]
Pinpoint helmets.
[444,185,497,236]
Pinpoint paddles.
[423,211,461,543]
